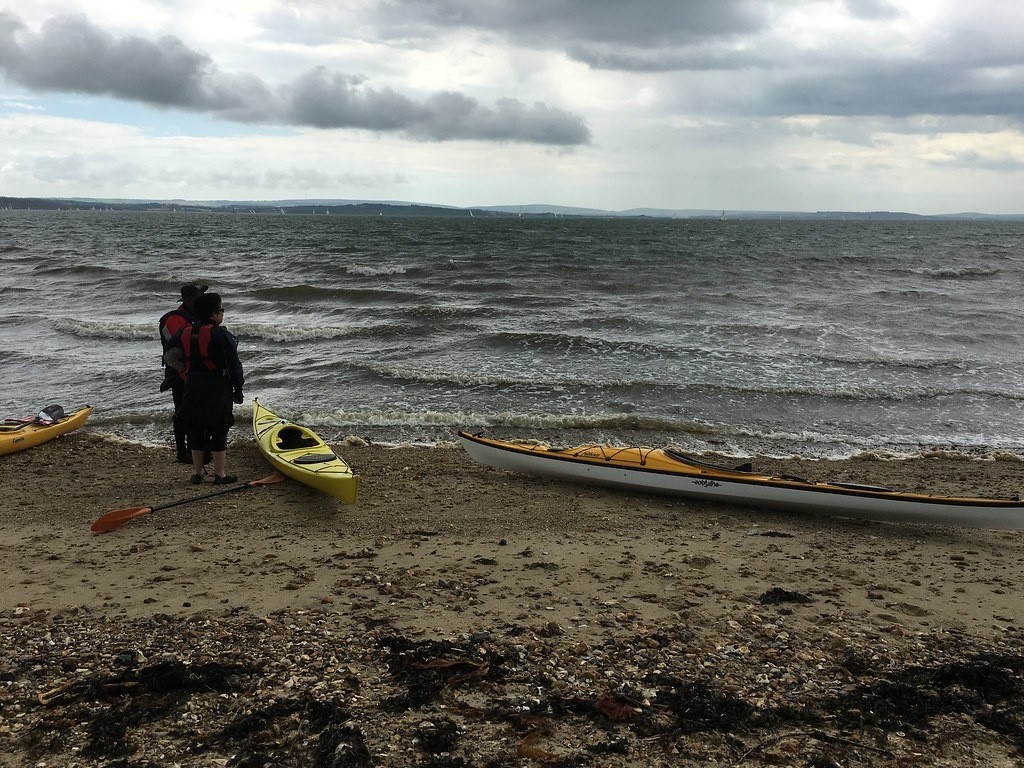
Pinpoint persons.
[163,292,244,483]
[160,284,211,464]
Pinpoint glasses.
[216,308,225,312]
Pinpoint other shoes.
[177,456,193,464]
[213,472,237,484]
[191,474,203,485]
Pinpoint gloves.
[233,390,244,404]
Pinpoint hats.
[176,282,209,302]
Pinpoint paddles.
[7,404,64,431]
[90,474,287,532]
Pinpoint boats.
[457,429,1024,533]
[0,402,95,458]
[251,396,363,505]
